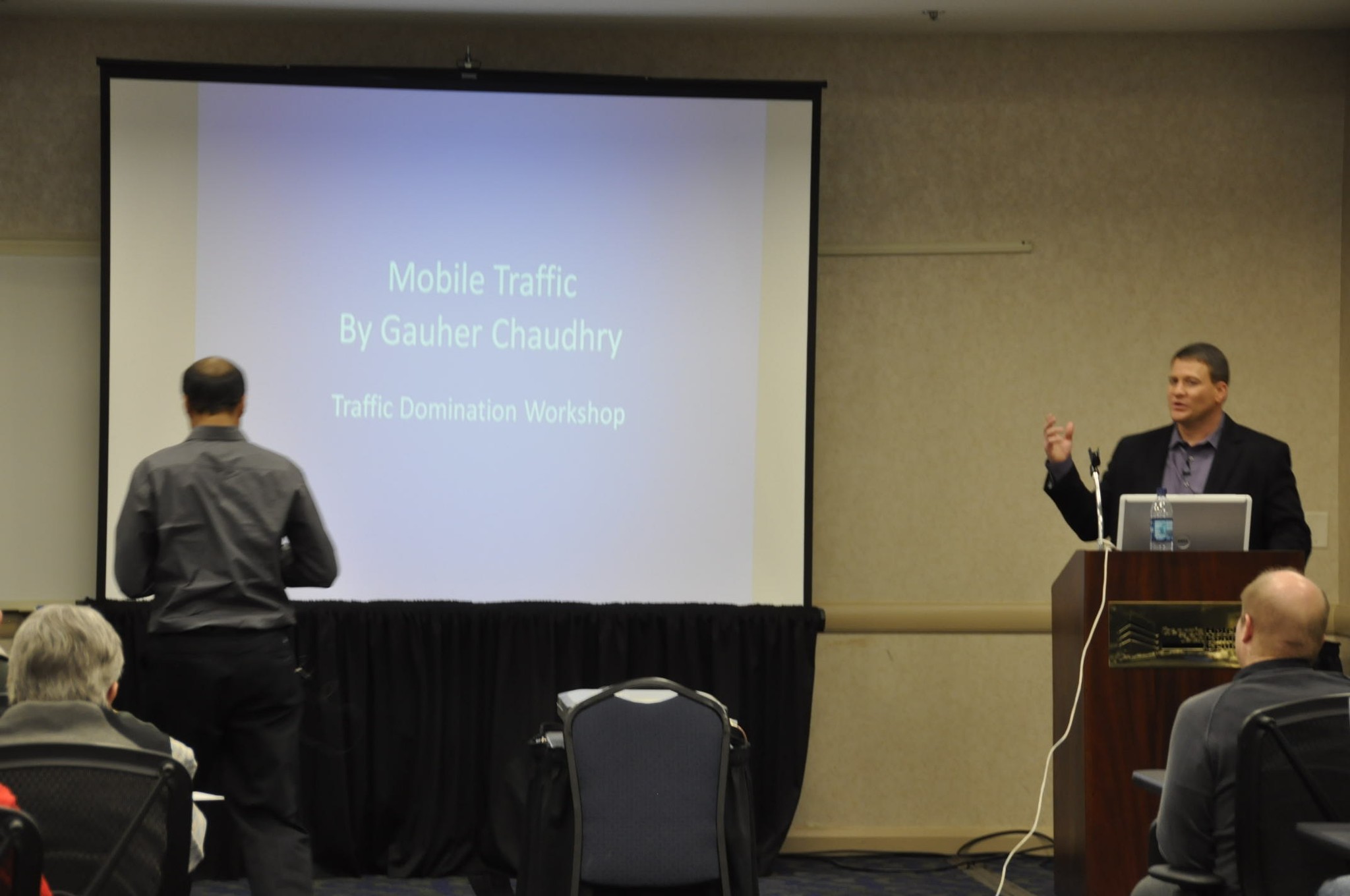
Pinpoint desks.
[75,596,832,874]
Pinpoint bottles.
[1149,487,1174,551]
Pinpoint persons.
[0,603,207,875]
[1039,341,1312,578]
[1126,568,1350,896]
[116,356,337,896]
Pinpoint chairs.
[0,739,195,896]
[562,673,734,896]
[1147,691,1350,896]
